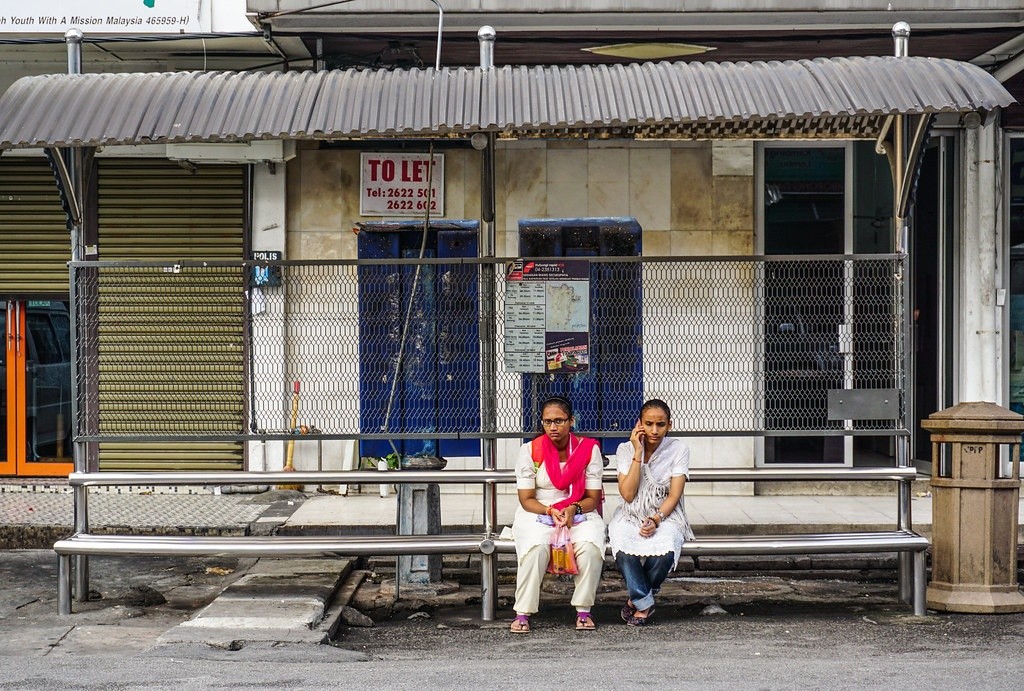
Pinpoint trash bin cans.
[920,401,1024,613]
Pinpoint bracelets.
[656,511,666,521]
[570,501,583,515]
[632,458,642,463]
[648,516,658,528]
[546,507,551,514]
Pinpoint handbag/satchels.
[545,522,578,575]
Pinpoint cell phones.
[635,419,644,445]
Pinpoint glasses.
[541,418,569,426]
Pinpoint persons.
[510,396,605,633]
[609,398,696,627]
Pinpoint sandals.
[575,613,596,630]
[510,615,530,633]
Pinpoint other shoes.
[627,606,655,626]
[621,599,637,622]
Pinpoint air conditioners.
[167,139,285,166]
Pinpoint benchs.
[53,465,931,622]
